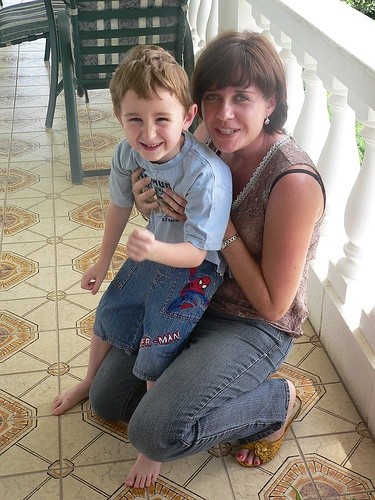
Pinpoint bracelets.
[220,233,241,251]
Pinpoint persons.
[52,44,232,489]
[130,31,327,468]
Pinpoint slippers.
[236,395,302,467]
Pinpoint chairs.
[0,0,199,186]
